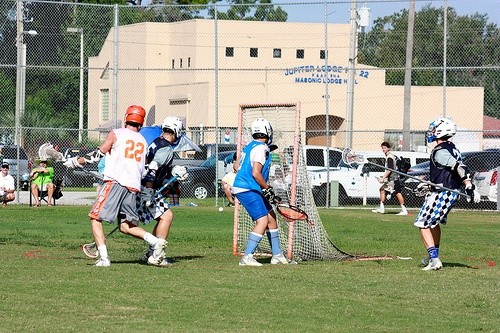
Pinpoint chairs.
[29,166,64,206]
[160,176,182,206]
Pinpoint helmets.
[161,116,186,138]
[123,105,146,128]
[430,117,457,138]
[250,118,274,139]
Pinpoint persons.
[63,104,169,268]
[0,161,16,208]
[47,141,65,199]
[411,117,483,272]
[0,145,5,167]
[221,149,299,207]
[29,156,55,208]
[370,142,410,216]
[136,116,190,268]
[229,117,298,267]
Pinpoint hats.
[1,162,9,168]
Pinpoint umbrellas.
[138,125,203,155]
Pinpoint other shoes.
[239,203,243,207]
[34,205,41,207]
[226,203,234,207]
[47,203,52,206]
[2,203,6,207]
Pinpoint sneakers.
[152,238,167,260]
[238,254,263,266]
[93,258,110,267]
[396,211,408,216]
[421,258,431,265]
[271,252,297,264]
[147,256,173,266]
[372,208,384,213]
[422,258,442,270]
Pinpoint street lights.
[16,29,39,205]
[66,27,84,143]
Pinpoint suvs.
[0,144,33,191]
[58,146,101,187]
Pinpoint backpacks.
[395,155,412,179]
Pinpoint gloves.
[140,187,154,207]
[62,155,85,170]
[262,186,282,203]
[414,181,432,197]
[171,165,189,181]
[464,182,481,203]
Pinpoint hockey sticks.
[266,192,308,223]
[342,147,472,198]
[82,166,187,258]
[38,142,103,181]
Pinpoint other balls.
[218,207,224,212]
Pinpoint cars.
[165,142,500,211]
